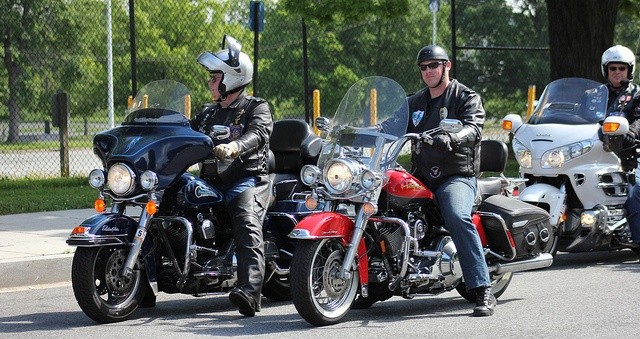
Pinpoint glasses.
[609,66,626,71]
[419,62,444,71]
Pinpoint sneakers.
[473,287,497,317]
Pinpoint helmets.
[601,44,636,77]
[416,45,449,65]
[196,35,255,101]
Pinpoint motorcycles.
[502,78,639,271]
[287,76,554,327]
[66,80,348,324]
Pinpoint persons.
[331,46,497,316]
[188,49,274,317]
[575,44,637,247]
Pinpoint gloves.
[620,129,635,145]
[431,135,451,152]
[213,142,240,161]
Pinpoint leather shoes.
[228,288,256,317]
